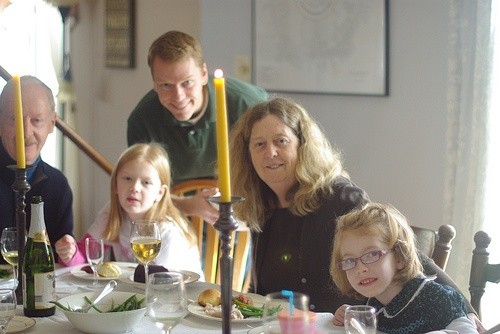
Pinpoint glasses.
[337,249,395,272]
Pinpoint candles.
[12,71,26,169]
[213,69,231,203]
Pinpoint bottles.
[17,195,57,318]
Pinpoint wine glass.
[0,227,29,291]
[84,238,104,288]
[146,271,189,334]
[129,220,162,313]
[0,288,17,334]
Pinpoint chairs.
[170,178,500,334]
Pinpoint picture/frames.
[251,0,391,97]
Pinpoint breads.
[198,288,222,307]
[133,263,171,284]
[98,262,120,277]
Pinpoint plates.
[247,326,268,333]
[127,269,200,292]
[6,316,36,334]
[187,299,283,323]
[69,261,139,281]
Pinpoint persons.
[331,203,478,334]
[55,143,205,282]
[128,32,270,280]
[0,76,74,265]
[228,97,486,333]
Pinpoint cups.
[261,290,311,334]
[344,305,377,334]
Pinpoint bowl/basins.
[55,292,151,334]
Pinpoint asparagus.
[233,299,283,317]
[48,295,147,313]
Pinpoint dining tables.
[0,261,385,334]
